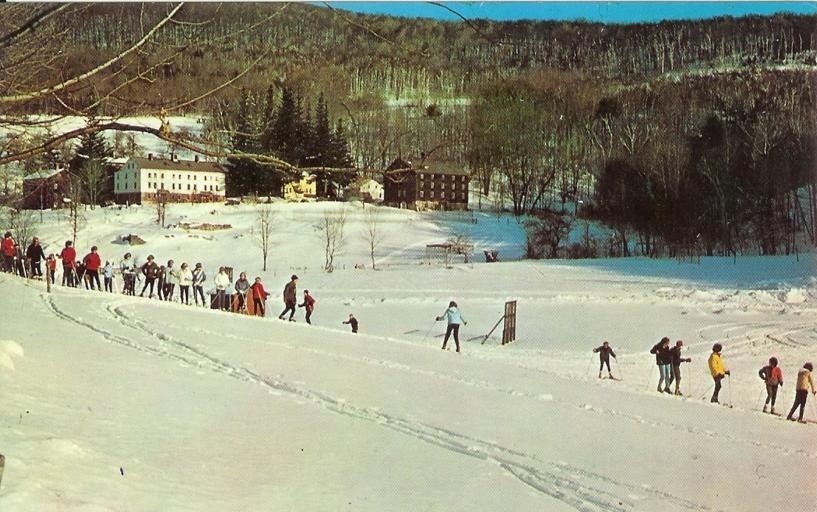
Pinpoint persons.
[787,363,816,424]
[593,341,617,379]
[708,344,731,403]
[650,337,674,395]
[435,300,467,352]
[2,232,116,293]
[279,275,314,324]
[342,313,359,333]
[759,357,783,415]
[215,266,270,318]
[120,252,207,309]
[669,341,692,396]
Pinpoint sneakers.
[763,406,805,423]
[610,374,613,378]
[599,373,601,378]
[658,388,683,396]
[290,318,296,322]
[279,316,285,320]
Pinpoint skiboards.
[602,376,622,382]
[703,396,733,408]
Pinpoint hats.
[291,275,298,279]
[676,341,683,346]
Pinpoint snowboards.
[753,407,781,416]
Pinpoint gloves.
[726,371,730,375]
[780,382,783,386]
[686,358,691,362]
